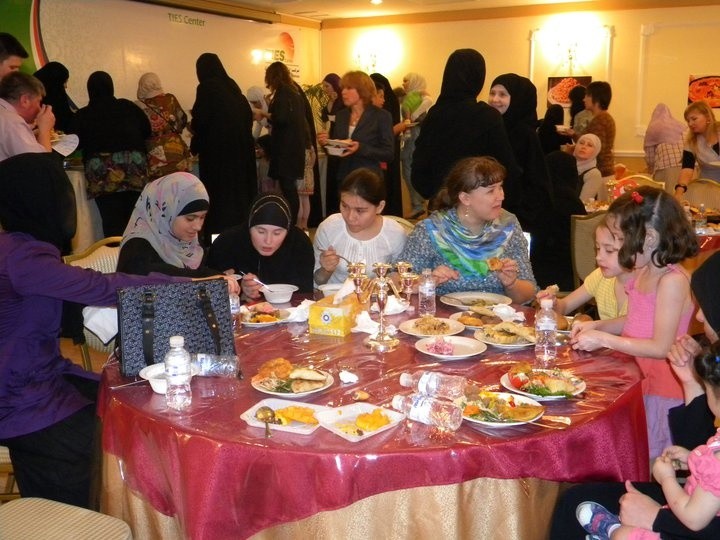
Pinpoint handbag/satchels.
[116,279,236,378]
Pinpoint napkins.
[289,299,314,322]
[352,311,380,335]
[494,304,526,323]
[372,294,409,315]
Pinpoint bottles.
[229,293,240,330]
[164,335,240,410]
[416,268,436,318]
[683,204,706,235]
[392,370,467,431]
[535,299,557,363]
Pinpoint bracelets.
[675,182,688,192]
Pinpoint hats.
[137,72,163,100]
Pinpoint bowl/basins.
[319,284,344,295]
[555,125,571,132]
[324,145,348,155]
[259,284,299,304]
[139,362,201,395]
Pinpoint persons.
[0,48,720,540]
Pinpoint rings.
[515,268,518,272]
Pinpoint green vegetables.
[522,385,574,399]
[469,410,514,423]
[269,378,294,393]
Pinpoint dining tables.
[97,292,648,540]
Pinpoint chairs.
[0,176,720,540]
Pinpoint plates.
[709,161,720,168]
[251,370,336,397]
[462,370,586,427]
[240,398,406,443]
[688,76,720,108]
[228,274,242,280]
[399,294,574,360]
[406,122,419,127]
[241,308,290,328]
[548,86,588,107]
[326,138,352,147]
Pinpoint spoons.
[258,407,275,440]
[317,247,355,264]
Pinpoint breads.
[249,314,277,323]
[459,305,592,344]
[269,406,319,425]
[289,367,329,393]
[257,358,294,378]
[486,257,504,271]
[356,408,391,432]
[544,284,560,294]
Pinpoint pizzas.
[688,77,720,107]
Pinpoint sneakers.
[576,501,621,540]
